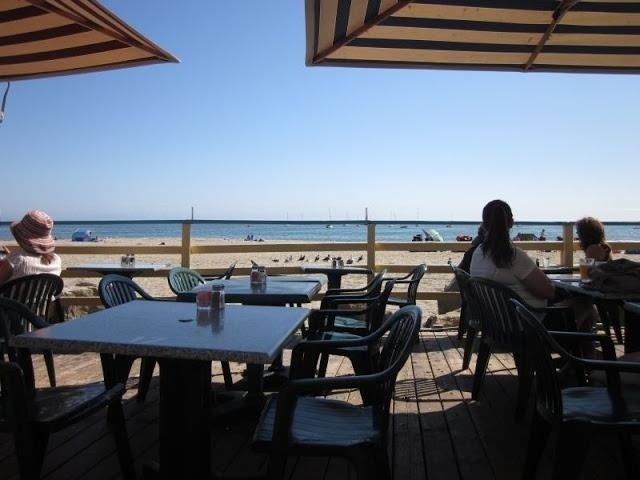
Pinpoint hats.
[9,212,56,255]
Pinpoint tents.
[71,228,93,241]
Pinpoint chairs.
[0,264,430,480]
[448,263,639,479]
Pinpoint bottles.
[259,270,267,283]
[212,284,221,309]
[121,253,136,267]
[219,283,225,309]
[534,255,549,267]
[251,266,259,284]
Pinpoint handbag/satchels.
[580,258,640,294]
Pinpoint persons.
[454,197,614,389]
[121,252,136,267]
[0,210,62,340]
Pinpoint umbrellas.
[304,0,640,76]
[0,0,181,83]
[422,228,444,242]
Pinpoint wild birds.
[448,258,452,264]
[272,255,363,266]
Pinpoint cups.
[580,258,595,283]
[195,287,211,311]
